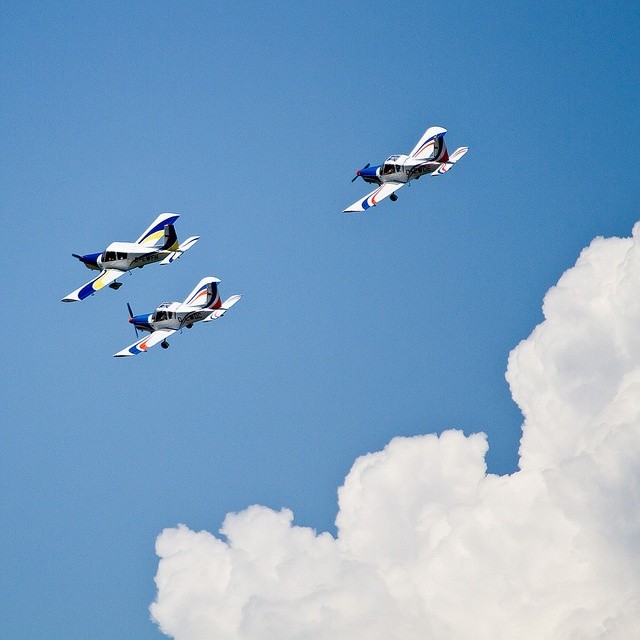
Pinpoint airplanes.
[64,214,199,302]
[343,127,465,211]
[116,277,240,357]
